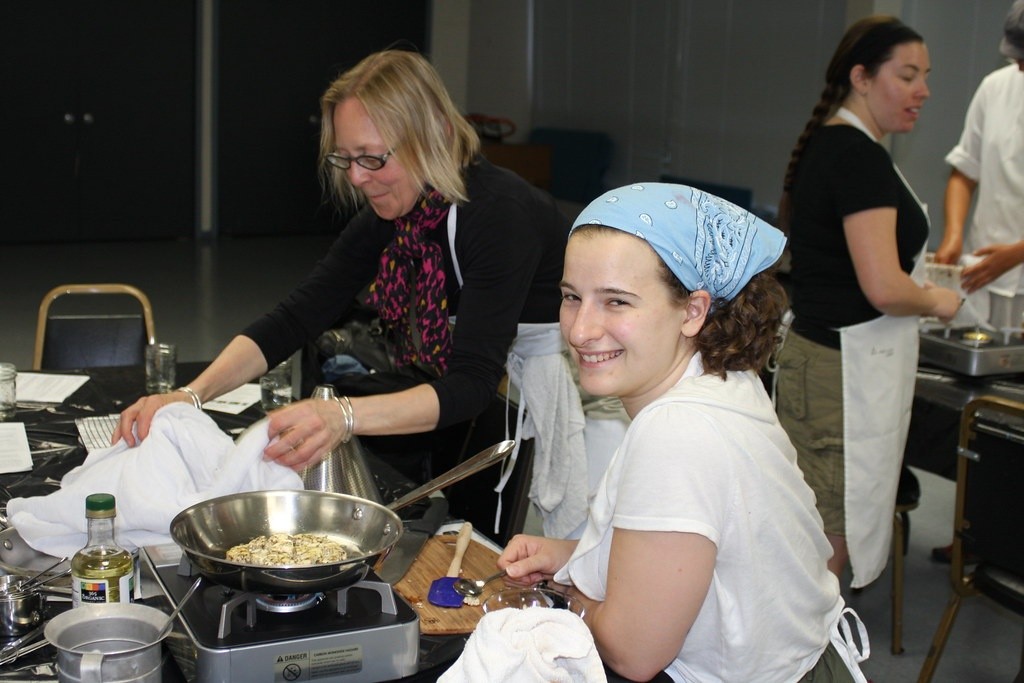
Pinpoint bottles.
[71,493,135,611]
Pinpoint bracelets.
[175,387,202,410]
[333,396,353,444]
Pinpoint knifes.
[377,497,448,587]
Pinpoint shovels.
[428,521,474,609]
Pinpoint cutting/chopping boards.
[370,532,550,635]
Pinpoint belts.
[790,316,842,351]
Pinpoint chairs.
[446,372,535,547]
[915,396,1024,683]
[892,466,921,656]
[33,283,157,371]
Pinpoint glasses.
[324,148,400,170]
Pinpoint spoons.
[452,570,507,596]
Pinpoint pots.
[0,513,73,593]
[169,439,516,597]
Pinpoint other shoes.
[931,544,952,564]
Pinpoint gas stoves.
[917,325,1023,377]
[135,543,419,683]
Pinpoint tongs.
[0,620,51,665]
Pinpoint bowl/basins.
[0,575,47,637]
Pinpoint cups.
[260,358,293,413]
[145,344,176,396]
[0,362,17,423]
[482,588,587,625]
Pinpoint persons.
[112,53,575,551]
[775,16,966,645]
[498,183,869,683]
[934,0,1024,328]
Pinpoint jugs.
[44,602,173,682]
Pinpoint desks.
[0,362,675,683]
